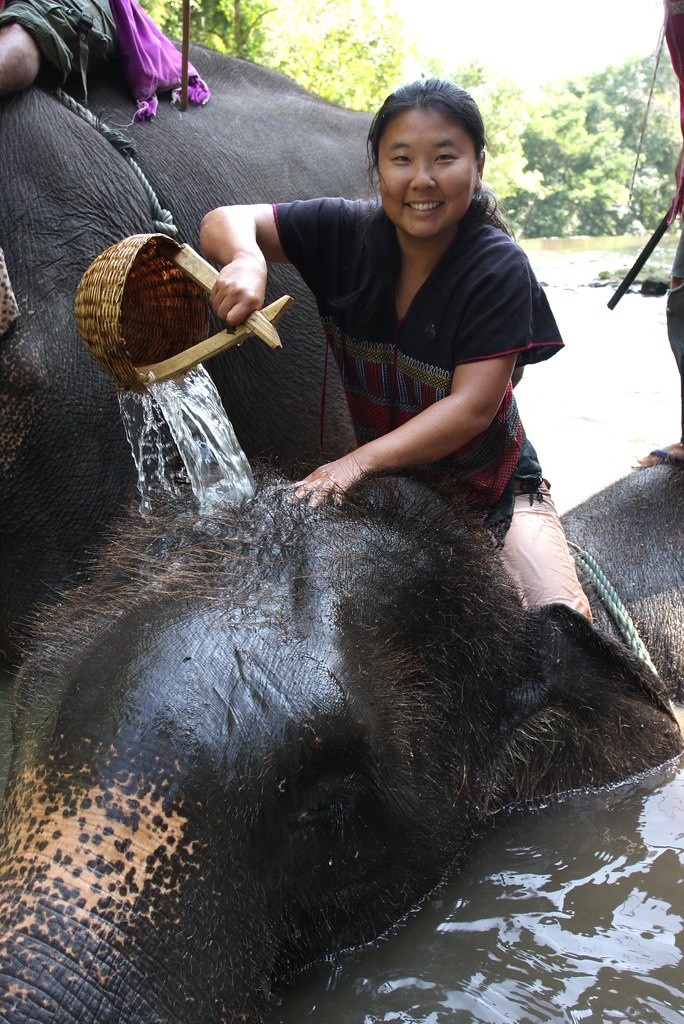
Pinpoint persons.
[0,0,118,100]
[632,0,684,469]
[196,79,595,624]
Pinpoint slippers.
[630,449,684,472]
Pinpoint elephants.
[0,35,684,1024]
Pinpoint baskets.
[74,229,294,395]
